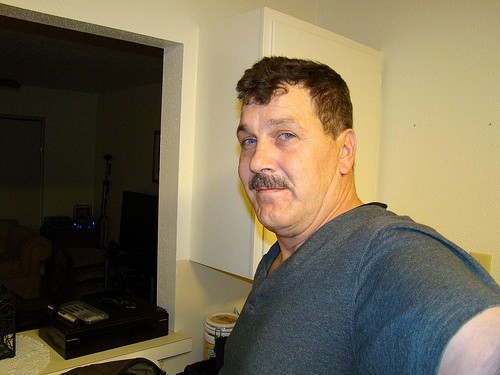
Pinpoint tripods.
[98,154,112,246]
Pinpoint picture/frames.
[73,205,92,222]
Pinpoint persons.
[217,57,500,375]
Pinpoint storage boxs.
[39,298,169,359]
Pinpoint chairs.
[53,247,109,299]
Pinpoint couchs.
[0,219,52,299]
